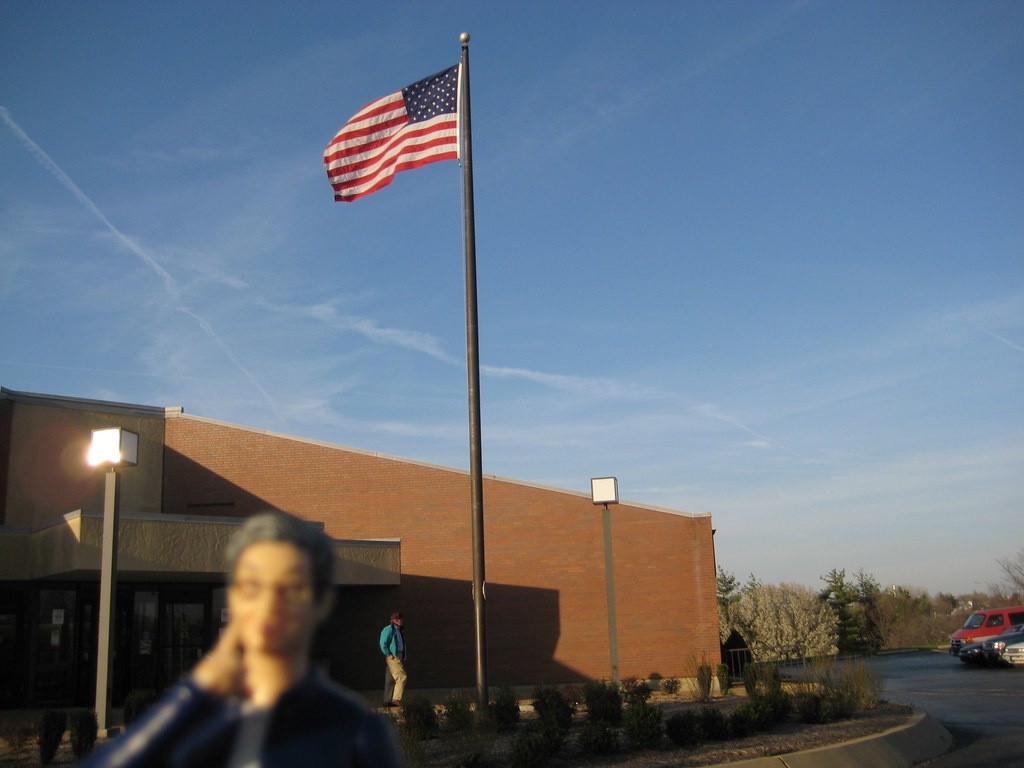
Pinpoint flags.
[322,63,463,205]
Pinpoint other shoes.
[383,700,401,707]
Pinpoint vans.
[948,604,1024,660]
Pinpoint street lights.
[86,426,140,740]
[590,476,621,708]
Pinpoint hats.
[392,612,403,619]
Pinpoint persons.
[79,511,408,767]
[379,611,408,709]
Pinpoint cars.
[956,624,1024,669]
[983,626,1024,669]
